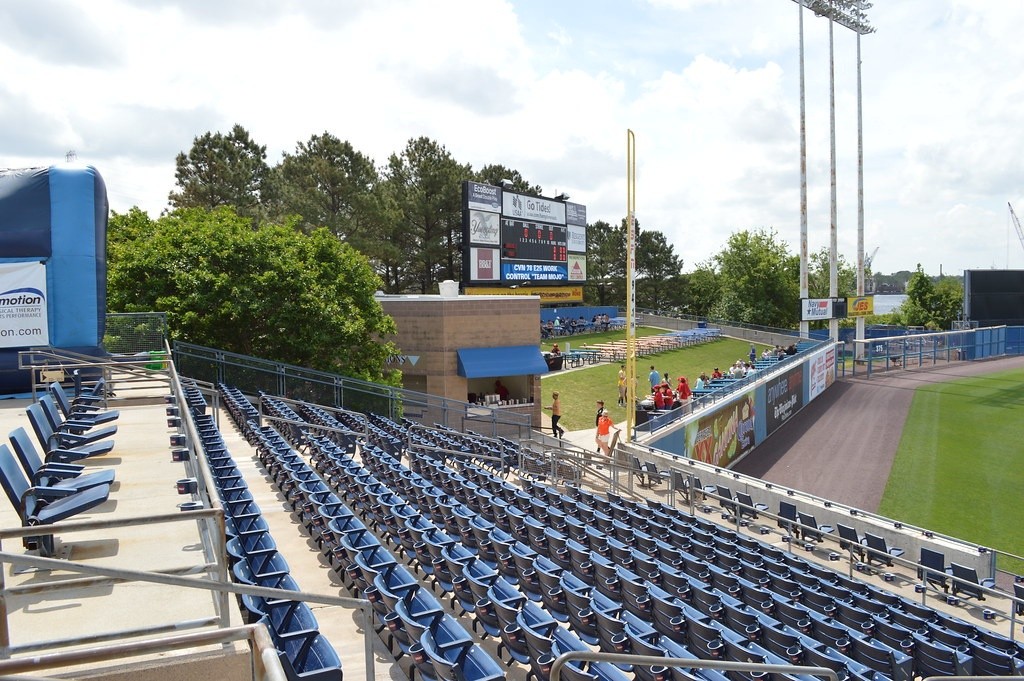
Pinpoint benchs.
[554,315,638,333]
[559,327,721,369]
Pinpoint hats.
[680,376,685,381]
[661,384,667,387]
[601,409,609,417]
[651,384,661,389]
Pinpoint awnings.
[456,344,549,377]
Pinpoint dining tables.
[570,348,600,364]
[690,341,821,393]
[579,345,619,350]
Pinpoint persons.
[592,313,609,332]
[595,400,611,453]
[495,379,510,405]
[646,386,665,415]
[618,365,640,407]
[552,344,560,355]
[545,392,564,438]
[539,315,587,344]
[657,304,704,321]
[762,348,772,361]
[661,372,673,392]
[711,367,721,379]
[694,371,709,397]
[676,375,692,414]
[662,384,674,410]
[773,344,798,360]
[747,343,757,363]
[729,358,756,379]
[648,365,660,393]
[598,409,619,464]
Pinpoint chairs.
[164,378,1024,681]
[0,367,119,554]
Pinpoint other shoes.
[559,431,564,436]
[616,400,627,407]
[553,432,558,438]
[596,448,600,452]
[635,395,638,399]
